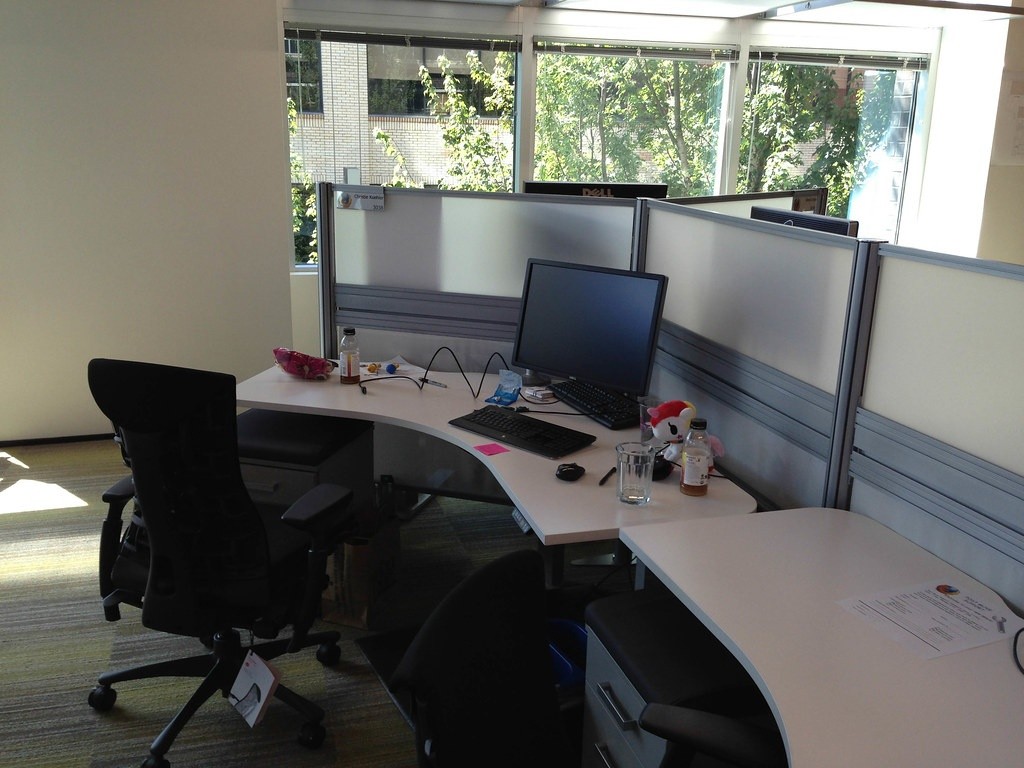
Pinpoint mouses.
[556,462,585,481]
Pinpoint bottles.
[339,327,360,385]
[679,417,711,496]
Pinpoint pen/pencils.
[419,377,448,389]
[598,467,617,485]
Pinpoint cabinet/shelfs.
[582,591,757,768]
[237,408,372,526]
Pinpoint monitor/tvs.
[523,181,669,199]
[751,206,859,238]
[511,258,669,401]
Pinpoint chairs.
[86,358,343,768]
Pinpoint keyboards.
[545,379,647,429]
[448,405,597,460]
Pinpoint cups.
[615,442,655,506]
[639,398,668,446]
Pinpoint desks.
[234,358,757,768]
[584,507,1024,768]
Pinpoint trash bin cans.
[544,616,588,714]
